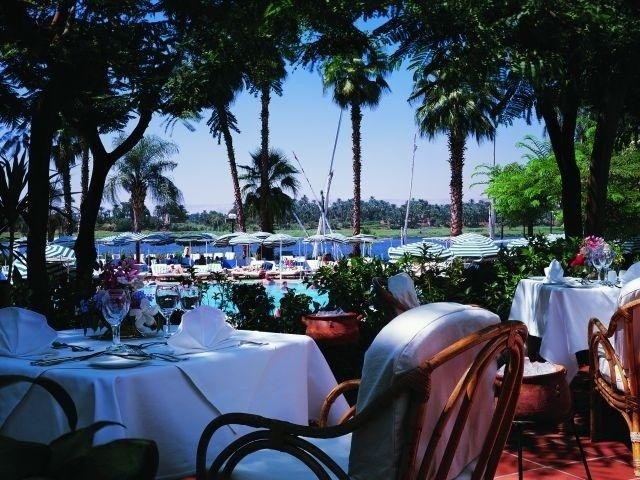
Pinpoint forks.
[51,343,83,354]
[140,353,191,364]
[134,343,170,350]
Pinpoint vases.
[98,304,144,338]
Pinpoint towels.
[0,307,58,359]
[167,304,239,354]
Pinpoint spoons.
[125,341,165,347]
[57,343,96,352]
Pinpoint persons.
[281,282,292,291]
[308,280,322,289]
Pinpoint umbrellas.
[387,231,567,263]
[96,231,384,267]
[0,235,76,277]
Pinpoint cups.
[608,270,617,286]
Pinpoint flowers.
[78,257,167,335]
[571,237,622,263]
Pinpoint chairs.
[195,303,528,480]
[372,271,419,326]
[0,374,159,479]
[583,277,640,480]
[96,254,371,279]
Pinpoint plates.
[91,352,145,371]
[564,282,582,288]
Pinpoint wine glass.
[592,258,614,284]
[102,289,131,348]
[156,284,196,342]
